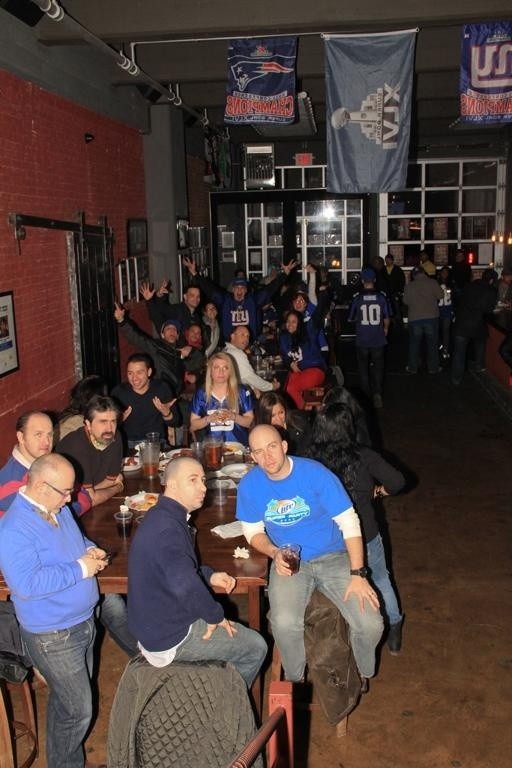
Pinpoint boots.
[389,621,403,656]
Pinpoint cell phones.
[100,550,115,564]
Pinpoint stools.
[1,367,348,768]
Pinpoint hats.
[361,269,375,281]
[232,279,247,288]
[161,320,181,340]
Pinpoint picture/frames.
[0,290,22,377]
[176,220,236,303]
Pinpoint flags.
[223,35,299,126]
[320,28,419,194]
[459,21,512,126]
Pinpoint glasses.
[43,482,74,498]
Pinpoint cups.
[189,442,207,473]
[203,437,224,471]
[279,543,301,574]
[253,354,276,379]
[211,481,230,504]
[146,432,166,451]
[139,442,160,479]
[114,511,134,537]
[243,447,255,463]
[136,515,144,527]
[189,528,198,550]
[216,403,229,422]
[158,472,166,493]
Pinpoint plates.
[121,441,167,471]
[158,459,173,472]
[125,493,161,511]
[165,449,193,460]
[222,441,243,456]
[221,463,254,479]
